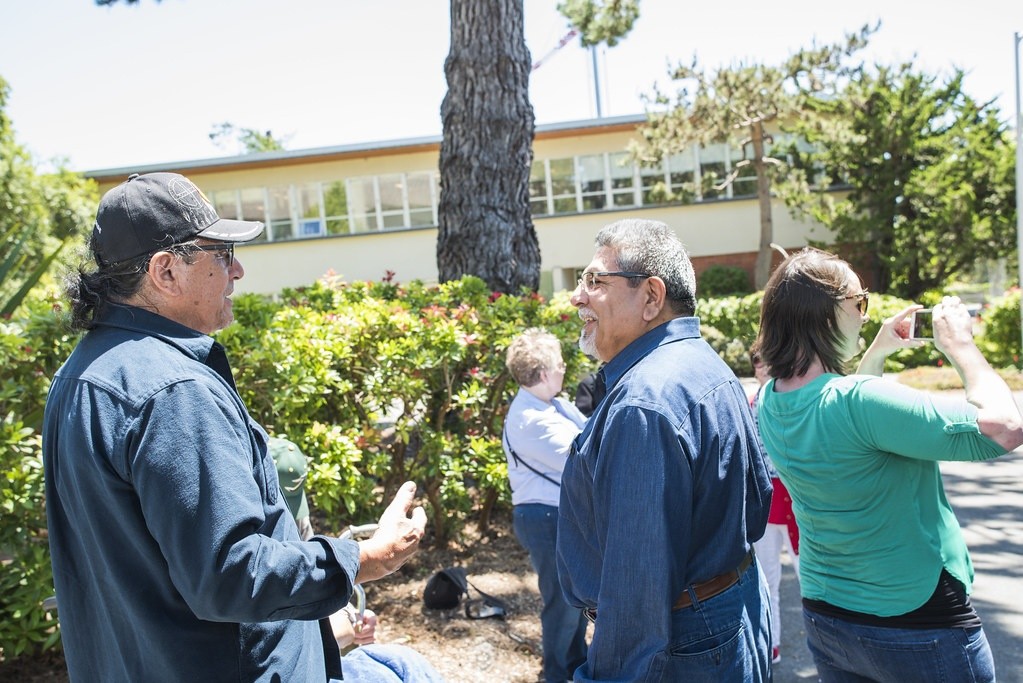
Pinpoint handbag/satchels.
[422,565,505,619]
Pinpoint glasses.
[168,241,235,267]
[577,270,652,293]
[834,291,870,316]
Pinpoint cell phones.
[351,583,366,633]
[909,308,936,342]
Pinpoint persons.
[501,326,591,683]
[41,171,429,683]
[746,334,800,665]
[755,249,1023,683]
[555,218,774,683]
[576,360,606,419]
[265,437,446,683]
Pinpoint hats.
[93,171,265,267]
[267,437,309,522]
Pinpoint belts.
[584,550,754,623]
